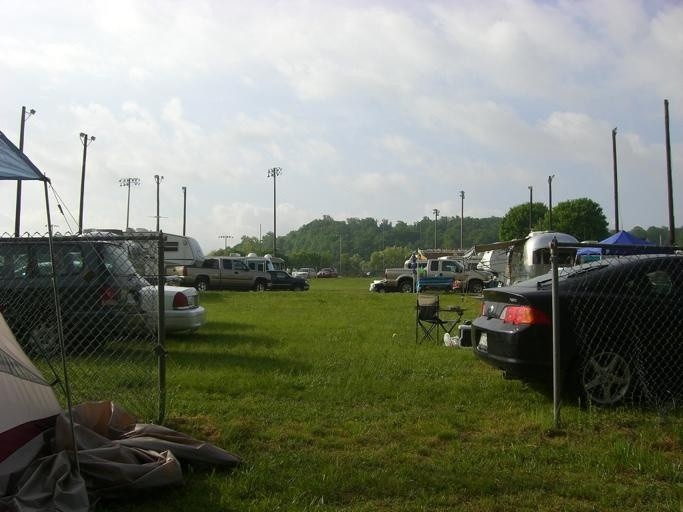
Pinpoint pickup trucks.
[384,259,487,294]
[166,256,269,292]
[138,272,194,287]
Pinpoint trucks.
[476,230,578,287]
[78,227,204,272]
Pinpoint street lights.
[118,177,141,233]
[13,104,35,236]
[181,186,186,236]
[77,129,96,236]
[152,173,166,231]
[432,208,439,249]
[267,166,282,257]
[335,231,342,276]
[217,236,234,257]
[459,189,465,250]
[611,126,620,234]
[546,174,556,230]
[527,185,533,233]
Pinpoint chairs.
[416,295,464,345]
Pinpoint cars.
[369,274,413,293]
[265,270,309,292]
[470,252,681,410]
[14,259,205,353]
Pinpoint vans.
[298,268,315,278]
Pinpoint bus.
[224,257,274,273]
[403,256,482,270]
[268,257,286,270]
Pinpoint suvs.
[0,236,146,359]
[316,268,337,278]
